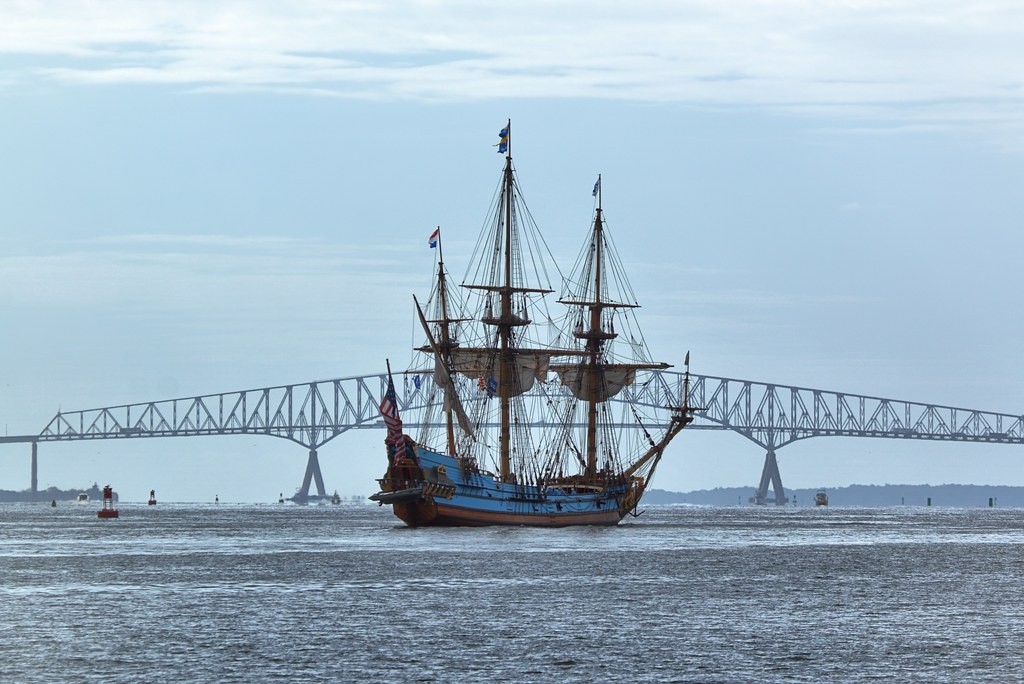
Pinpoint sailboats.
[367,118,709,528]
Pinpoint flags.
[378,358,407,468]
[591,179,599,197]
[684,351,689,365]
[492,122,511,155]
[414,375,420,389]
[487,377,498,393]
[428,229,439,249]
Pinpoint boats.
[331,490,341,504]
[148,489,157,505]
[97,484,118,518]
[814,489,828,506]
[77,493,89,505]
